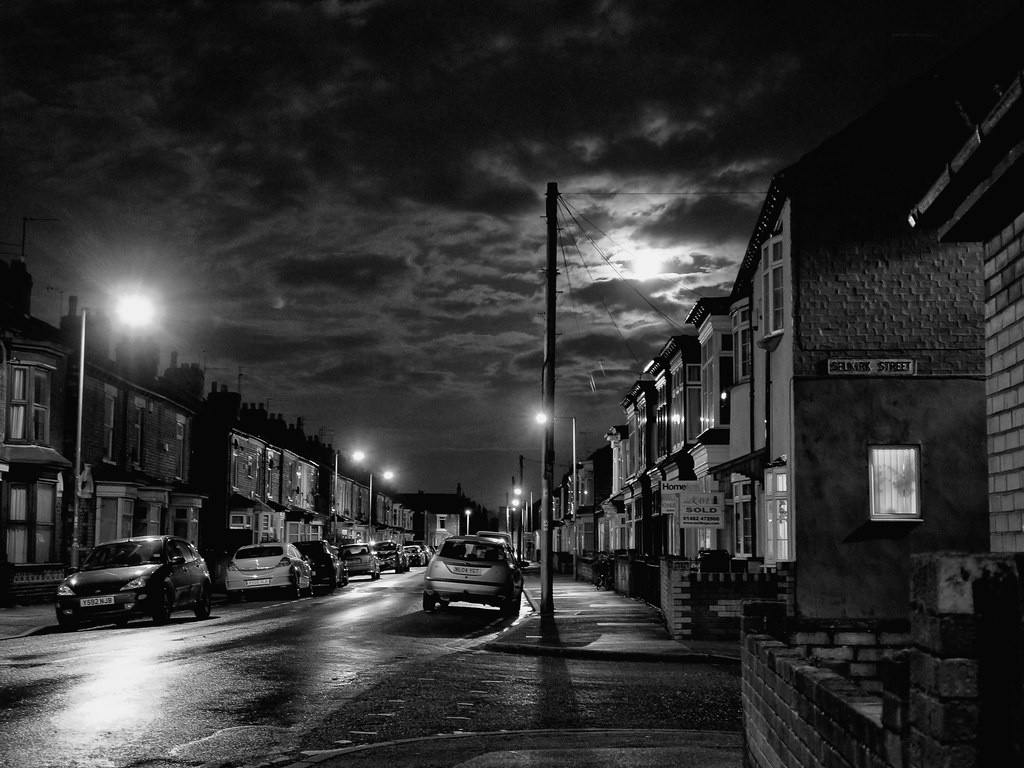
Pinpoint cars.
[292,540,344,593]
[423,535,530,615]
[330,546,349,587]
[225,542,314,602]
[54,534,212,630]
[372,531,511,574]
[340,544,380,579]
